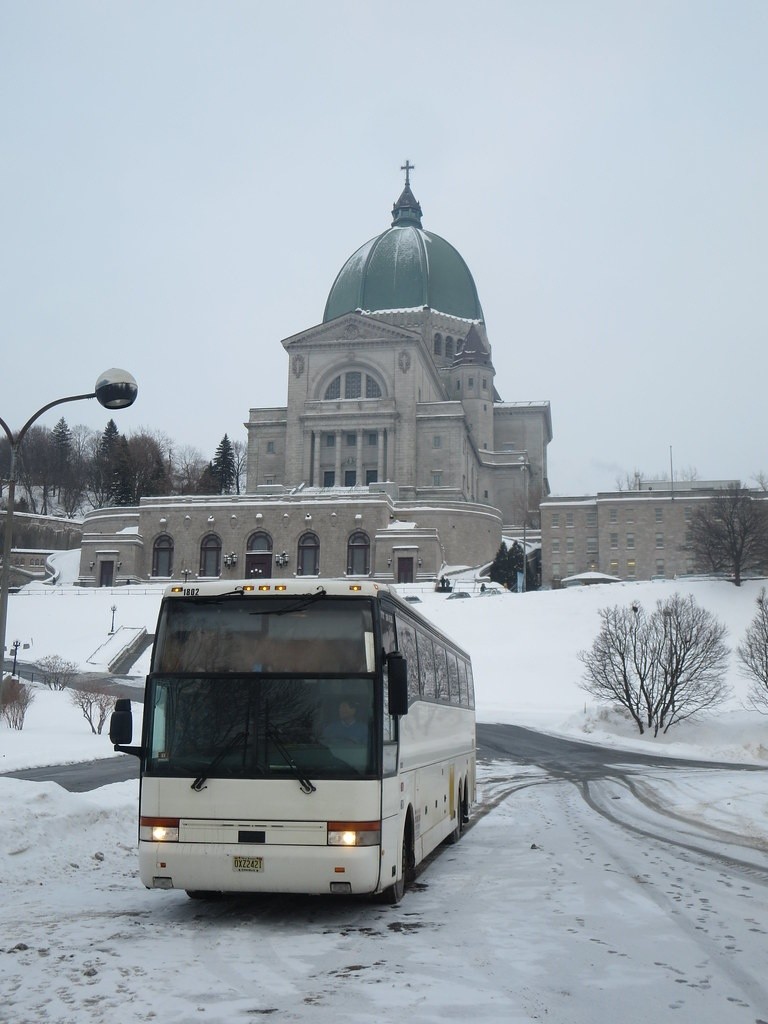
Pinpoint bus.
[110,578,480,907]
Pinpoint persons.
[481,583,485,592]
[318,701,369,744]
[440,576,450,589]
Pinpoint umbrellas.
[561,571,622,583]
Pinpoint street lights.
[0,368,139,700]
